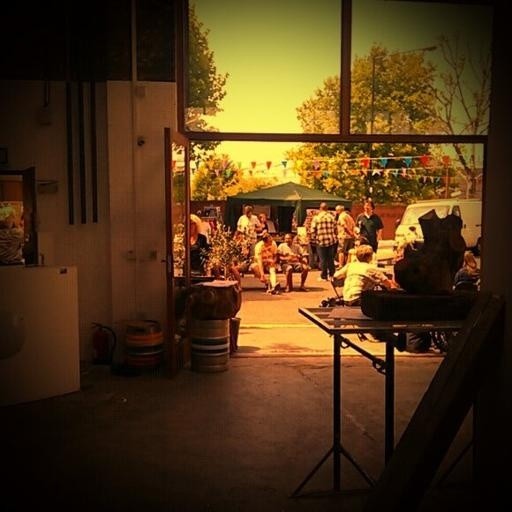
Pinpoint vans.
[393,198,482,259]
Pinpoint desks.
[292,306,463,496]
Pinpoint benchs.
[241,254,310,289]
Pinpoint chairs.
[331,277,368,341]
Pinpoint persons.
[330,243,401,308]
[0,207,17,229]
[472,236,482,256]
[187,192,384,295]
[452,248,482,291]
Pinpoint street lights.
[365,45,439,201]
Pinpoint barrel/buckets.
[231,318,241,351]
[187,319,231,372]
[127,320,162,369]
